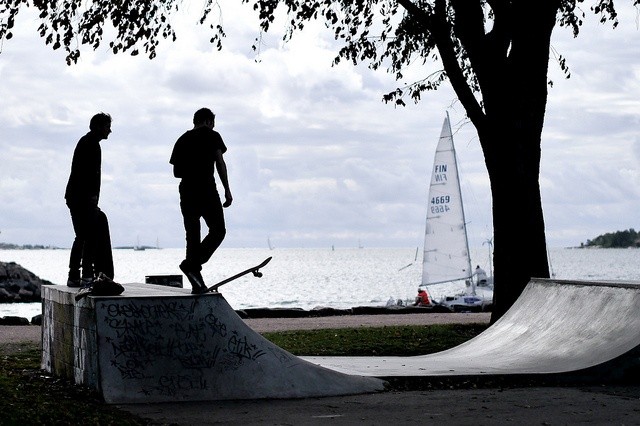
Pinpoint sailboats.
[419,109,493,314]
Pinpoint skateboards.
[208,256,272,292]
[95,211,114,280]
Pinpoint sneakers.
[180,260,208,290]
[80,278,94,287]
[67,278,82,287]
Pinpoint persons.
[64,112,113,286]
[169,107,233,294]
[472,266,487,282]
[413,288,429,306]
[462,269,471,286]
